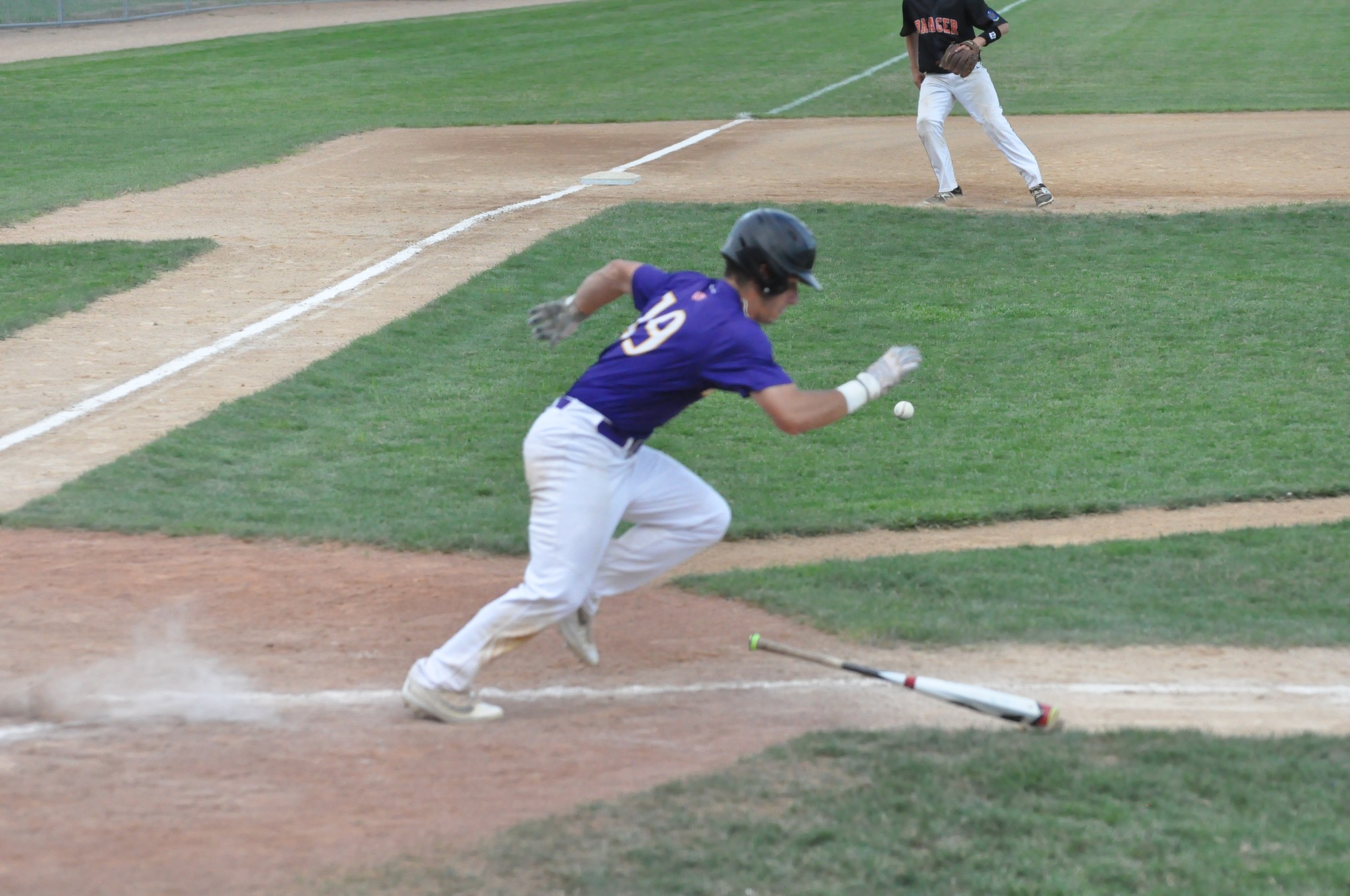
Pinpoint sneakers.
[560,609,599,664]
[401,679,502,722]
[924,186,963,205]
[1030,185,1054,206]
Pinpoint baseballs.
[894,401,914,420]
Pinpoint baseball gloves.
[935,40,982,78]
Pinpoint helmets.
[721,207,823,291]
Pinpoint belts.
[558,396,642,453]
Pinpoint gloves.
[858,344,921,396]
[527,296,583,343]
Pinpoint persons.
[899,0,1054,209]
[401,209,923,723]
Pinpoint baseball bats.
[748,633,1056,732]
